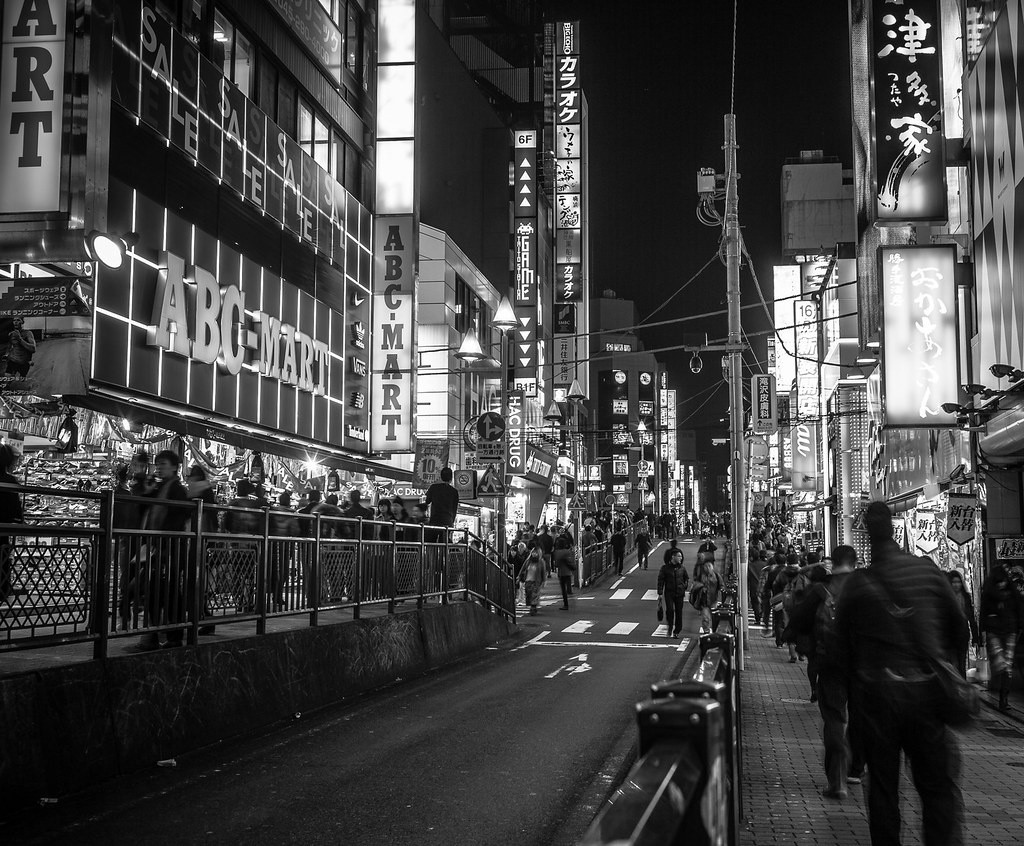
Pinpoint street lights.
[542,378,586,545]
[453,293,521,570]
[637,420,648,511]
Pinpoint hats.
[510,545,517,552]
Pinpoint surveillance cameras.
[950,465,965,480]
[690,356,703,373]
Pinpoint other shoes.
[702,625,710,633]
[612,570,624,577]
[118,615,221,656]
[637,565,648,571]
[817,761,867,799]
[667,632,680,639]
[747,621,808,663]
[546,566,560,579]
[530,608,537,616]
[559,606,569,610]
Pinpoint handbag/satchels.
[657,597,665,623]
[770,594,784,612]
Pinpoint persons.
[647,506,731,540]
[449,538,491,611]
[608,526,627,576]
[507,515,577,616]
[425,467,459,543]
[223,475,429,614]
[582,505,647,555]
[113,449,219,653]
[747,501,1024,846]
[634,526,653,571]
[657,535,726,639]
[0,433,25,603]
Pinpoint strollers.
[700,523,712,539]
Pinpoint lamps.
[989,363,1024,382]
[941,403,982,415]
[87,227,140,270]
[962,384,1005,400]
